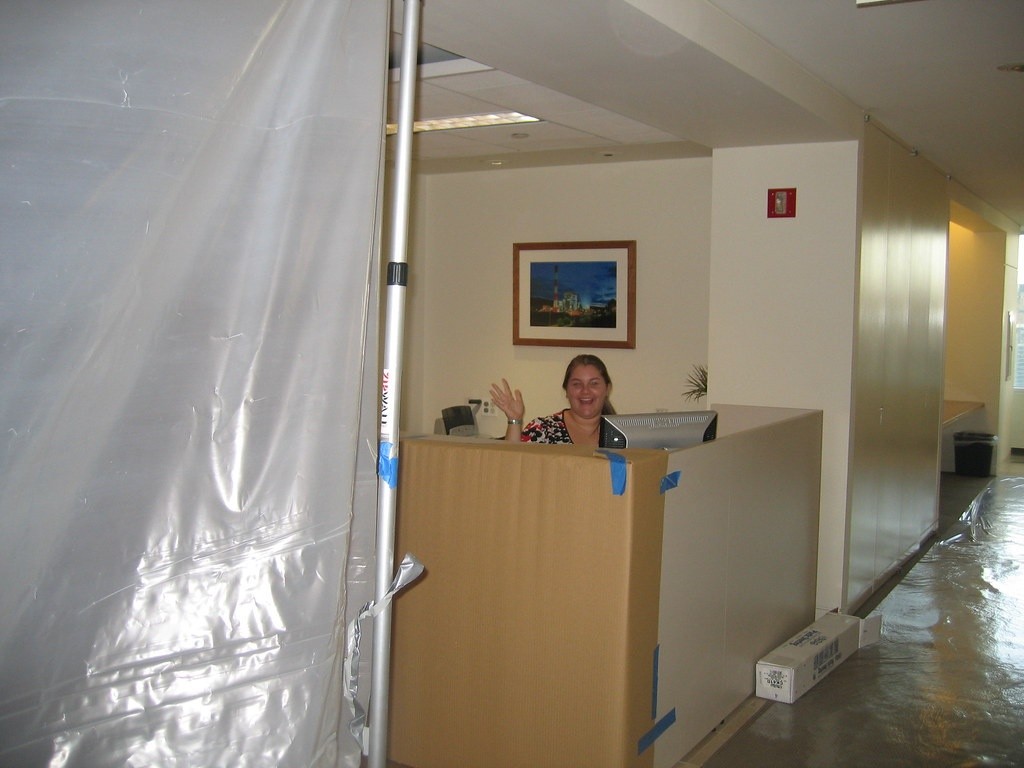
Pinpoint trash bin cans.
[952,431,996,478]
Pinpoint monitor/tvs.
[599,410,718,450]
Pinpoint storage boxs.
[756,607,884,704]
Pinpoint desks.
[387,404,824,768]
[465,394,498,416]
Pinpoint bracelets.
[507,419,521,425]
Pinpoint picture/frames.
[513,240,637,349]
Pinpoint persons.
[489,354,616,446]
